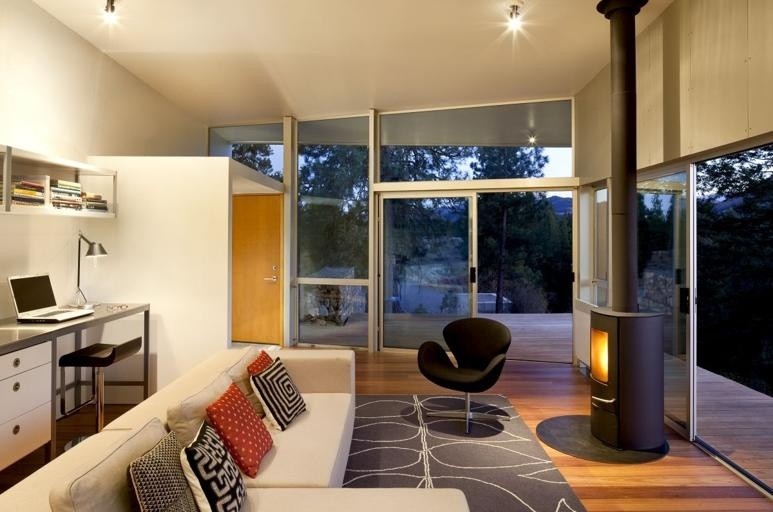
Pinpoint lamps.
[73,231,107,307]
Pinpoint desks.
[0,300,149,475]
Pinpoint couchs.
[0,346,467,512]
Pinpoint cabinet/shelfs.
[0,145,118,217]
[1,340,52,470]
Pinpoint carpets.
[346,395,585,512]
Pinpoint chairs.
[416,318,511,433]
[58,337,141,452]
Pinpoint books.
[0,177,107,211]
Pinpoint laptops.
[6,274,94,322]
